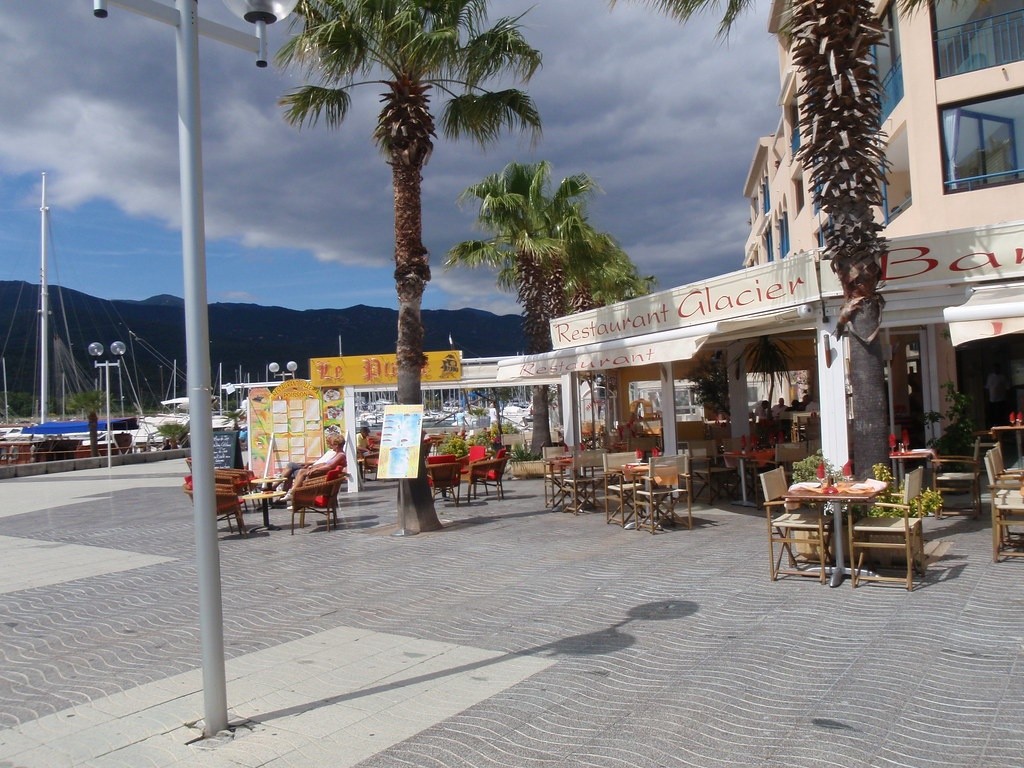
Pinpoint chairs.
[983,453,1024,563]
[184,484,248,539]
[759,465,834,585]
[456,452,492,499]
[633,454,693,535]
[987,446,1024,546]
[291,475,345,535]
[688,439,739,506]
[467,454,512,503]
[426,462,464,507]
[303,471,348,508]
[756,441,808,513]
[602,452,647,528]
[215,473,248,496]
[626,436,659,497]
[854,464,923,592]
[423,440,433,461]
[561,448,608,517]
[363,452,385,483]
[216,467,258,494]
[930,436,983,521]
[542,446,583,508]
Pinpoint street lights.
[87,341,126,470]
[91,0,306,737]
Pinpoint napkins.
[1009,412,1015,421]
[723,451,736,469]
[652,448,658,457]
[740,434,746,448]
[850,478,888,492]
[912,448,943,466]
[784,482,821,510]
[636,448,642,459]
[816,459,825,479]
[1016,412,1023,420]
[902,430,909,444]
[843,459,853,476]
[888,434,895,446]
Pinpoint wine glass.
[889,439,896,455]
[842,467,851,487]
[636,455,642,466]
[903,436,909,452]
[816,469,826,488]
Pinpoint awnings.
[943,282,1024,346]
[496,305,814,380]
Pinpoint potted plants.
[509,443,567,480]
[917,381,979,496]
[791,447,944,566]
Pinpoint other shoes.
[260,486,276,491]
[280,489,293,501]
[287,506,292,510]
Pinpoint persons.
[356,428,372,481]
[985,362,1011,441]
[260,432,347,502]
[754,395,819,447]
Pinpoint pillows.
[497,448,506,459]
[322,470,339,503]
[335,465,343,473]
[428,454,455,464]
[470,446,486,461]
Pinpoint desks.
[251,477,288,513]
[889,448,939,520]
[991,425,1024,469]
[242,491,286,534]
[541,458,595,513]
[620,464,669,531]
[720,452,761,507]
[782,480,892,588]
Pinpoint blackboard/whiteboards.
[214,430,238,468]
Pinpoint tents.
[22,417,137,434]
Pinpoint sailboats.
[1,171,344,466]
